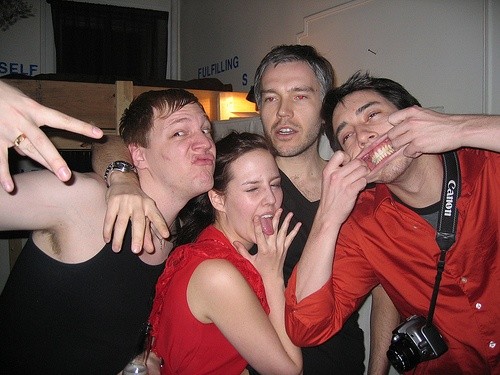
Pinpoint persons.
[145,130,302,375]
[0,80,217,375]
[283,72,500,375]
[90,44,365,375]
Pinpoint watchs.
[103,161,138,188]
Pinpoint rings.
[15,134,26,147]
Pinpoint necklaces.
[150,220,174,250]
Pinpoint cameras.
[387,314,448,372]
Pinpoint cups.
[121,359,148,375]
